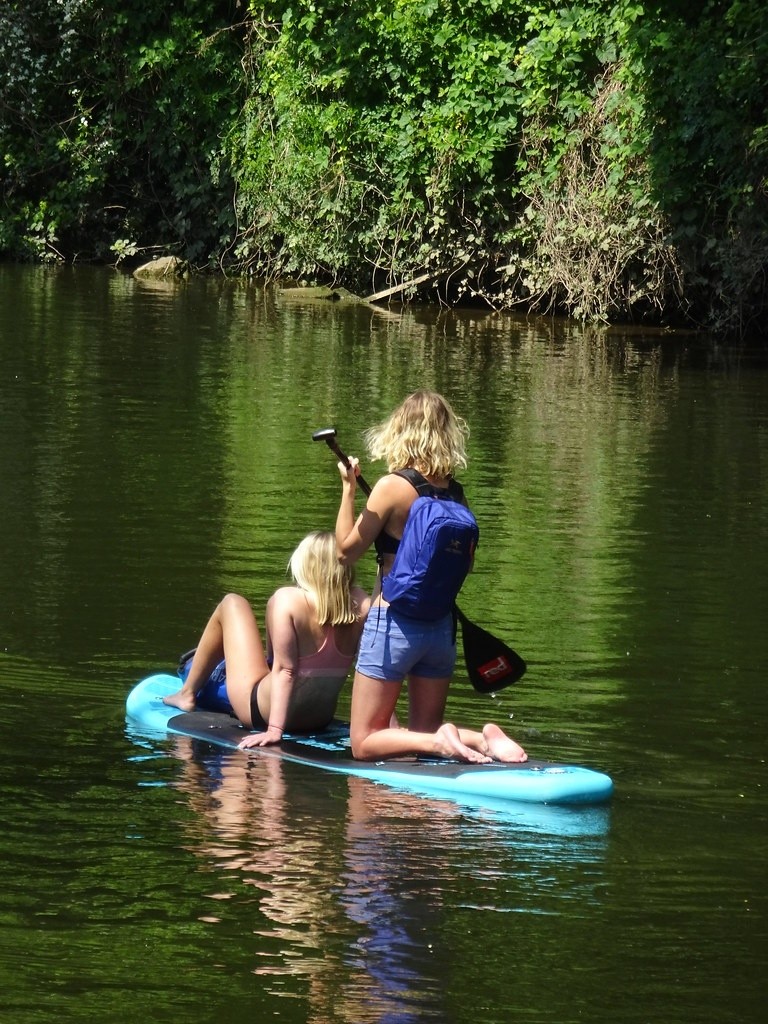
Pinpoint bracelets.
[268,724,284,731]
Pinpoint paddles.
[312,429,528,695]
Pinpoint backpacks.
[380,468,480,622]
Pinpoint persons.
[162,529,406,749]
[334,390,531,763]
[174,737,460,1023]
